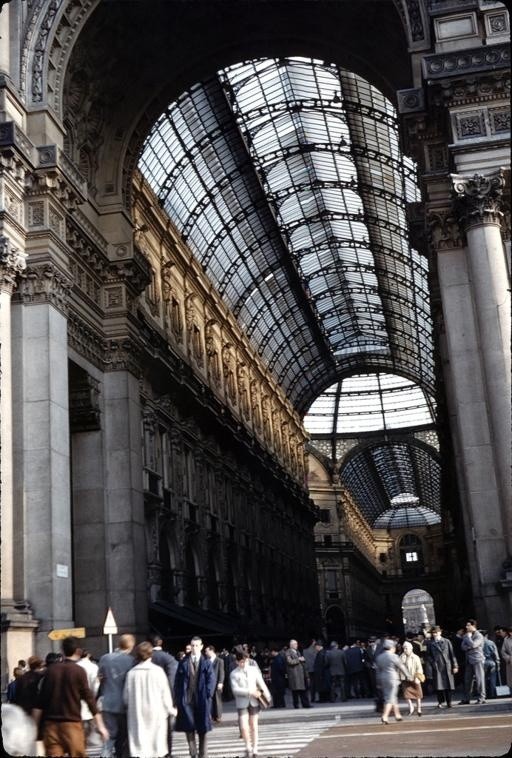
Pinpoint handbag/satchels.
[258,694,274,709]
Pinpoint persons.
[0,619,274,758]
[271,618,512,725]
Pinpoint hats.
[427,625,443,633]
